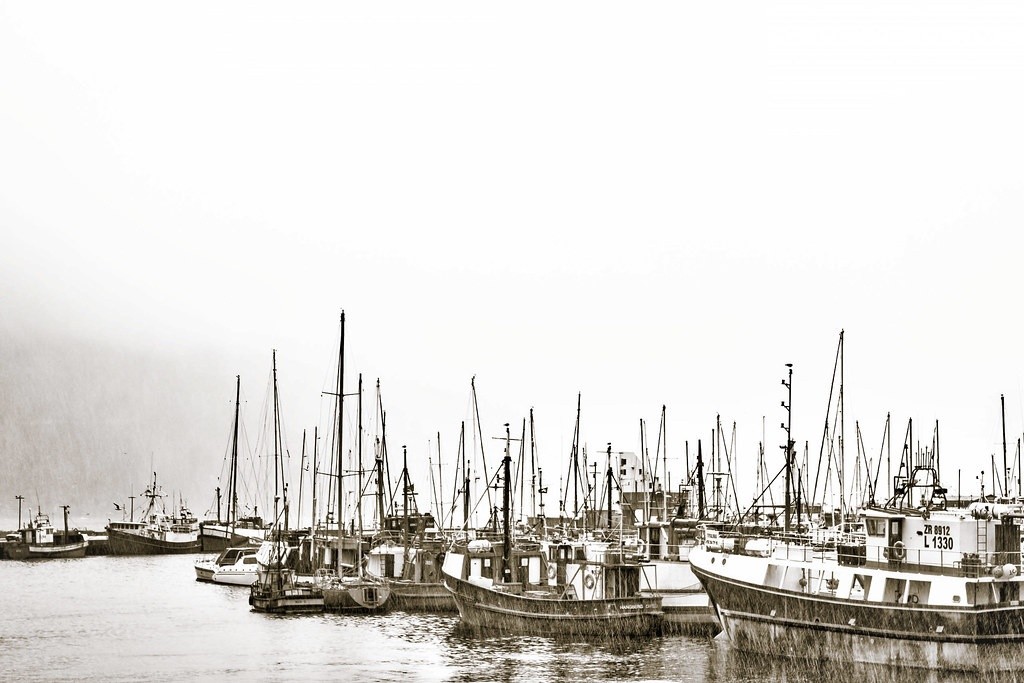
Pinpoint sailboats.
[195,308,1024,674]
[104,471,202,554]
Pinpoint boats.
[0,495,89,560]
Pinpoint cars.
[5,531,22,541]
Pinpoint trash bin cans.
[837,545,852,562]
[851,546,866,565]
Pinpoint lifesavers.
[893,541,906,560]
[584,574,594,589]
[547,565,556,580]
